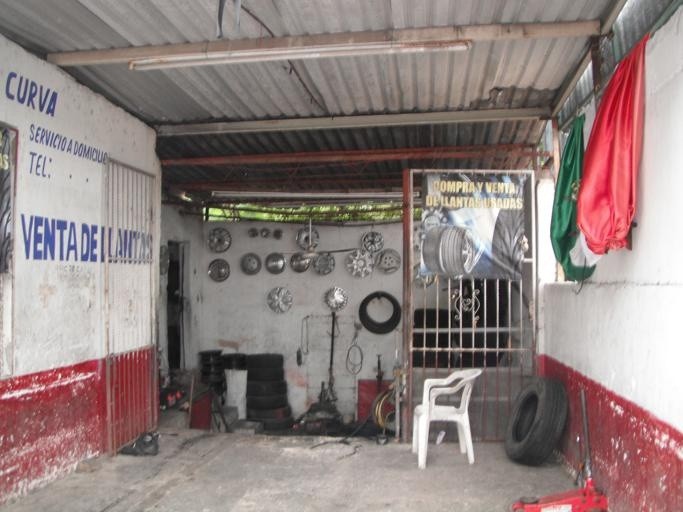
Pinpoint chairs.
[411,368,482,469]
